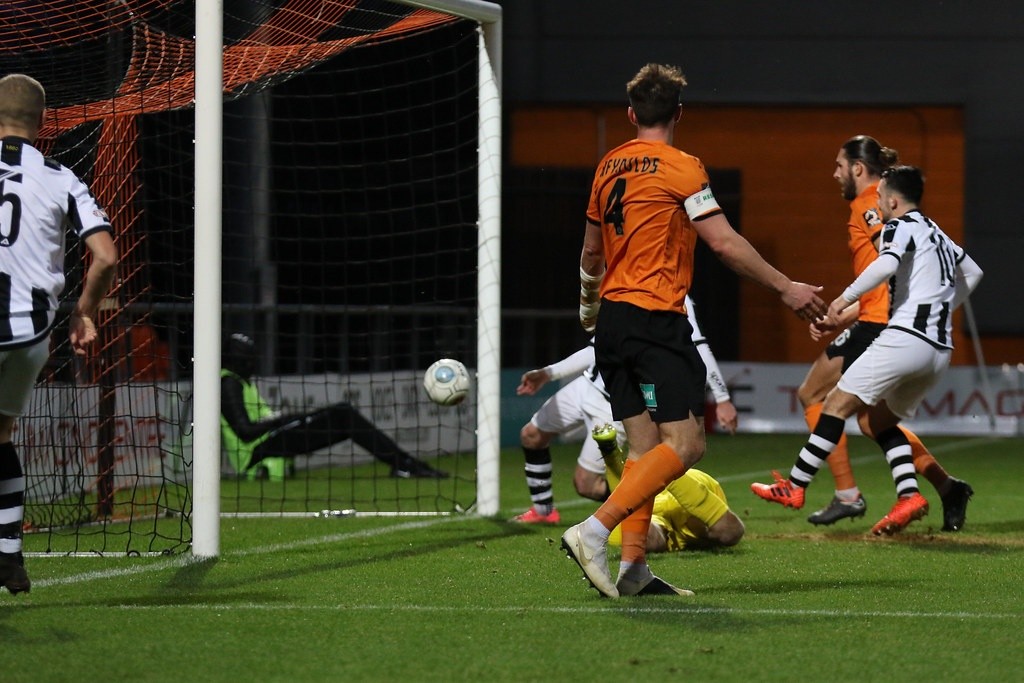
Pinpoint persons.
[0,74,117,599]
[510,136,984,550]
[561,63,829,603]
[114,311,450,476]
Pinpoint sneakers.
[751,472,805,510]
[872,491,928,535]
[807,493,866,526]
[558,525,620,598]
[592,423,619,454]
[942,477,974,532]
[615,566,695,598]
[508,507,559,524]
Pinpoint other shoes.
[0,566,29,593]
[395,460,448,479]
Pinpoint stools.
[246,455,296,483]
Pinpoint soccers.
[422,358,470,407]
